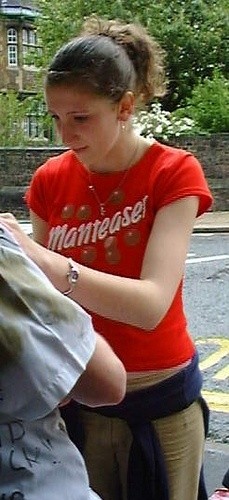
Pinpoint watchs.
[63,256,79,295]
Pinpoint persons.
[0,223,127,500]
[0,13,214,500]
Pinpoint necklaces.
[87,134,140,216]
